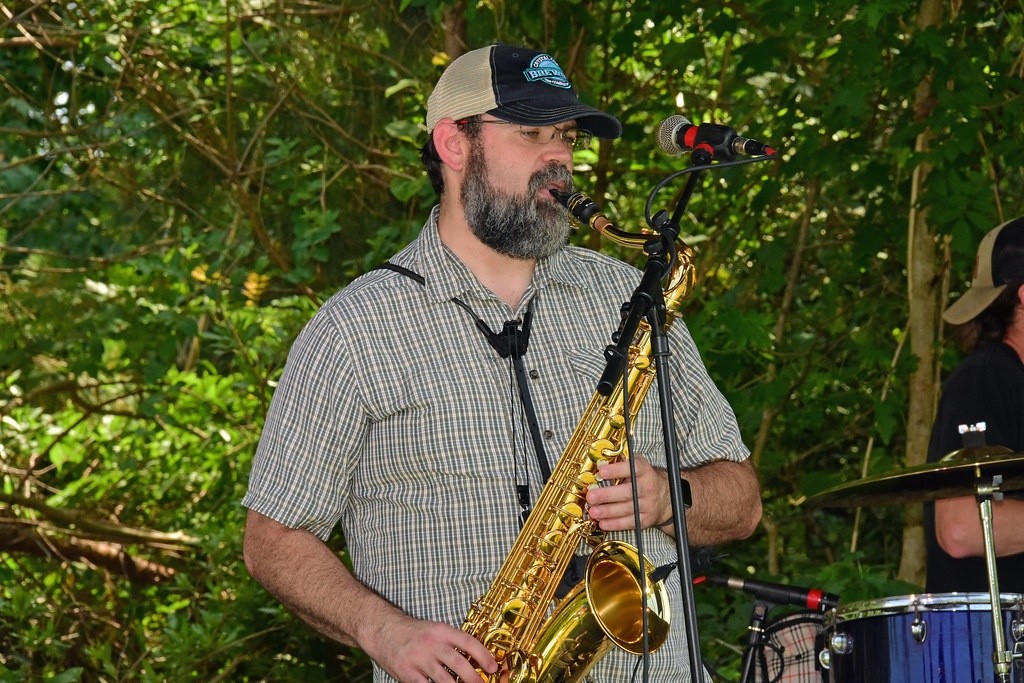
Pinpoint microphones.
[654,115,780,157]
[707,572,841,613]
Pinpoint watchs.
[659,479,693,528]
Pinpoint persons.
[919,216,1024,597]
[240,43,763,683]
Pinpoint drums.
[760,590,1024,683]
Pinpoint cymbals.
[803,435,1023,509]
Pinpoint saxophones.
[461,191,694,683]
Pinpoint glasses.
[455,120,593,152]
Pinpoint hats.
[941,216,1024,325]
[426,44,622,139]
